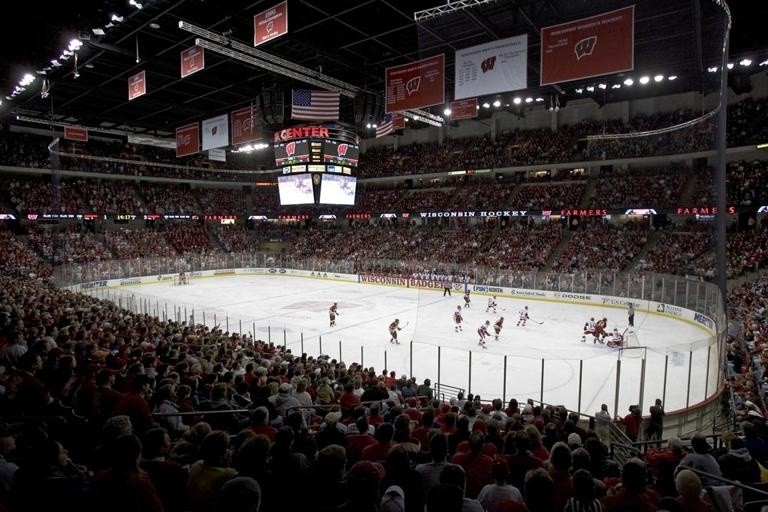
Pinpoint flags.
[291,89,340,119]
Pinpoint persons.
[1,95,768,512]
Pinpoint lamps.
[136,42,139,63]
[41,80,50,99]
[74,53,80,79]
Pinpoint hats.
[280,360,290,367]
[350,461,386,491]
[325,412,342,424]
[279,382,291,391]
[567,433,581,446]
[380,485,405,512]
